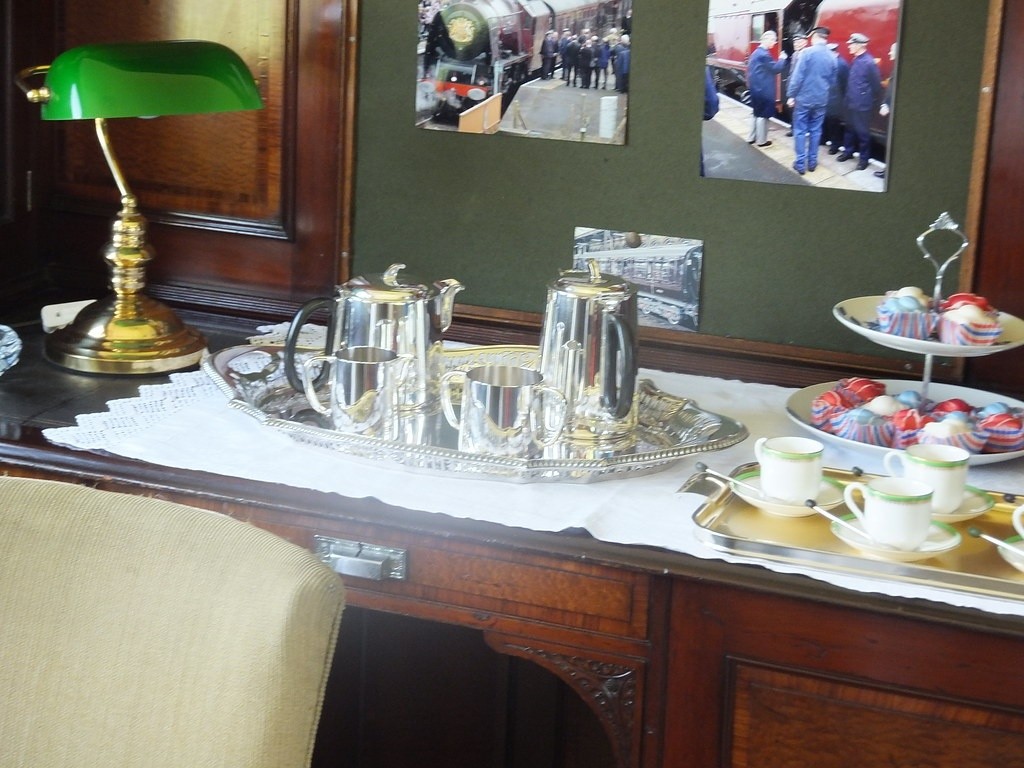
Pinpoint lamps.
[14,38,264,377]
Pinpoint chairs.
[0,470,344,768]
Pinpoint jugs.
[286,264,465,417]
[304,347,417,443]
[540,261,640,452]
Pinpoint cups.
[441,367,568,459]
[755,437,824,506]
[845,477,934,552]
[883,445,970,514]
[1013,503,1024,540]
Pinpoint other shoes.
[542,74,625,92]
[793,161,805,175]
[786,130,794,136]
[758,141,771,146]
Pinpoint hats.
[563,28,570,32]
[826,43,839,49]
[545,30,553,34]
[813,27,829,34]
[845,33,868,44]
[792,34,807,40]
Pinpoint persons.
[540,28,631,93]
[875,42,898,179]
[746,30,788,147]
[786,27,848,175]
[837,33,881,170]
[621,9,632,34]
[699,66,719,177]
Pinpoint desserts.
[812,376,1024,457]
[876,285,1005,347]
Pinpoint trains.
[707,0,902,143]
[573,244,702,329]
[416,0,633,122]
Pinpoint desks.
[0,277,1024,768]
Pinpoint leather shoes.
[873,171,885,178]
[836,153,852,162]
[828,146,837,154]
[856,159,868,170]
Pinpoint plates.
[997,535,1024,573]
[830,513,962,561]
[931,483,996,524]
[729,472,845,518]
